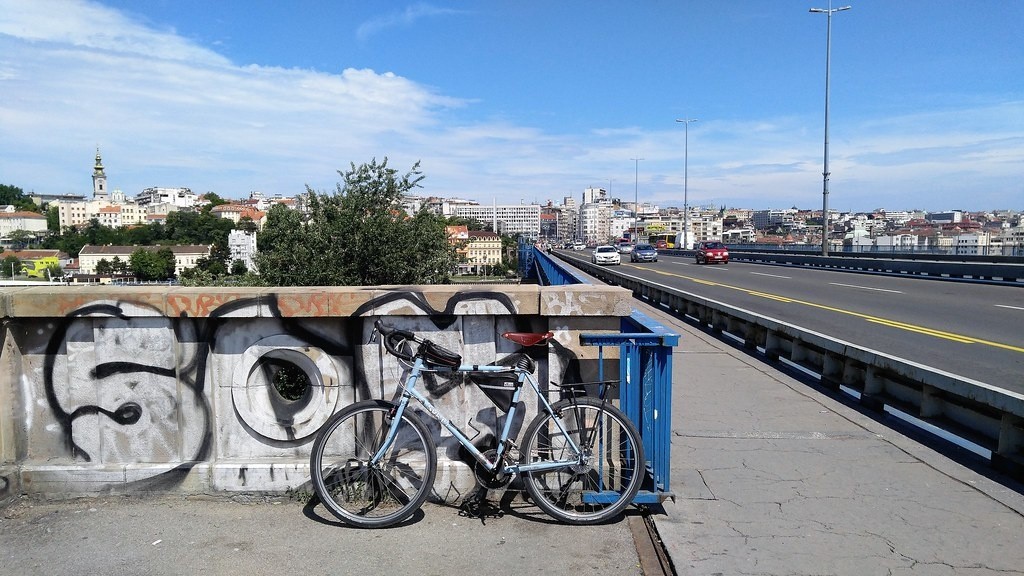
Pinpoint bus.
[616,237,629,248]
[648,232,676,248]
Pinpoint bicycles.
[308,317,646,530]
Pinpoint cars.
[615,242,633,254]
[695,240,730,265]
[591,245,621,265]
[656,239,667,249]
[630,244,659,262]
[540,238,587,251]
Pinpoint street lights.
[675,118,698,250]
[630,155,645,243]
[808,4,852,256]
[605,177,619,245]
